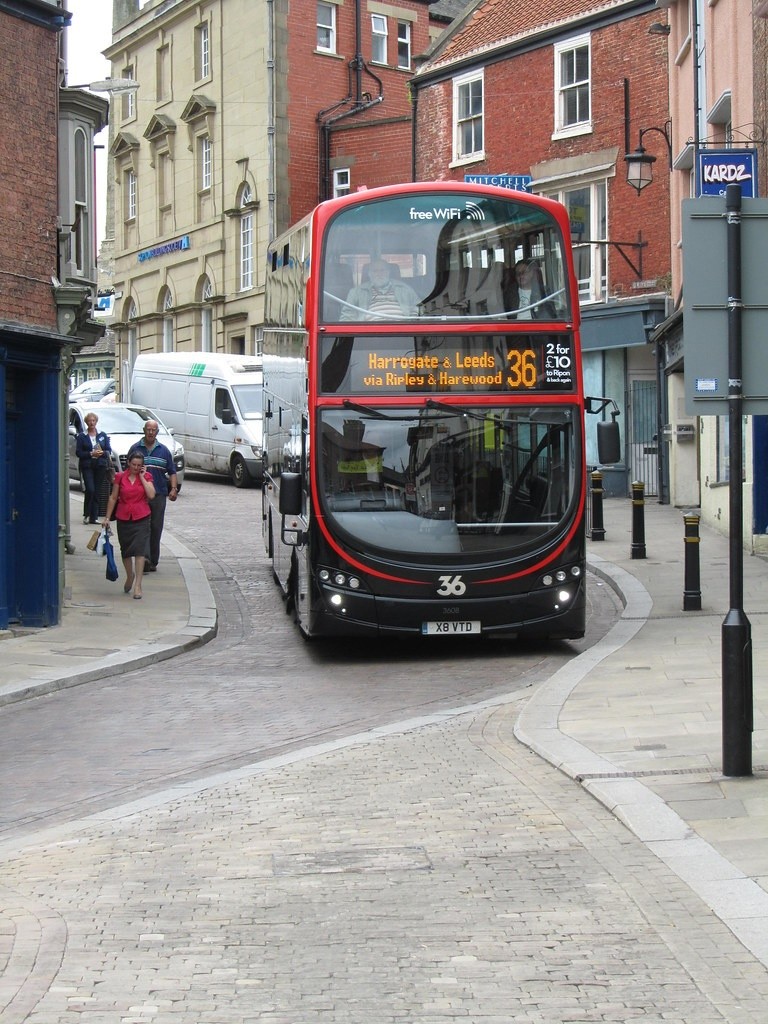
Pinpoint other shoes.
[144,561,155,572]
[88,517,102,524]
[133,586,142,599]
[84,514,90,524]
[124,572,135,593]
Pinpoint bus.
[260,182,622,640]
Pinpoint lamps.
[66,78,141,98]
[624,117,673,197]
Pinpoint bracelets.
[172,487,177,491]
[106,518,109,520]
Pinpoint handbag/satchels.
[86,525,118,582]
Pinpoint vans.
[130,351,263,489]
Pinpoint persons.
[101,450,156,599]
[75,412,111,524]
[337,431,405,493]
[339,259,424,321]
[125,420,177,575]
[499,259,553,319]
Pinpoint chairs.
[325,261,356,325]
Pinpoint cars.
[69,377,116,402]
[68,401,186,493]
